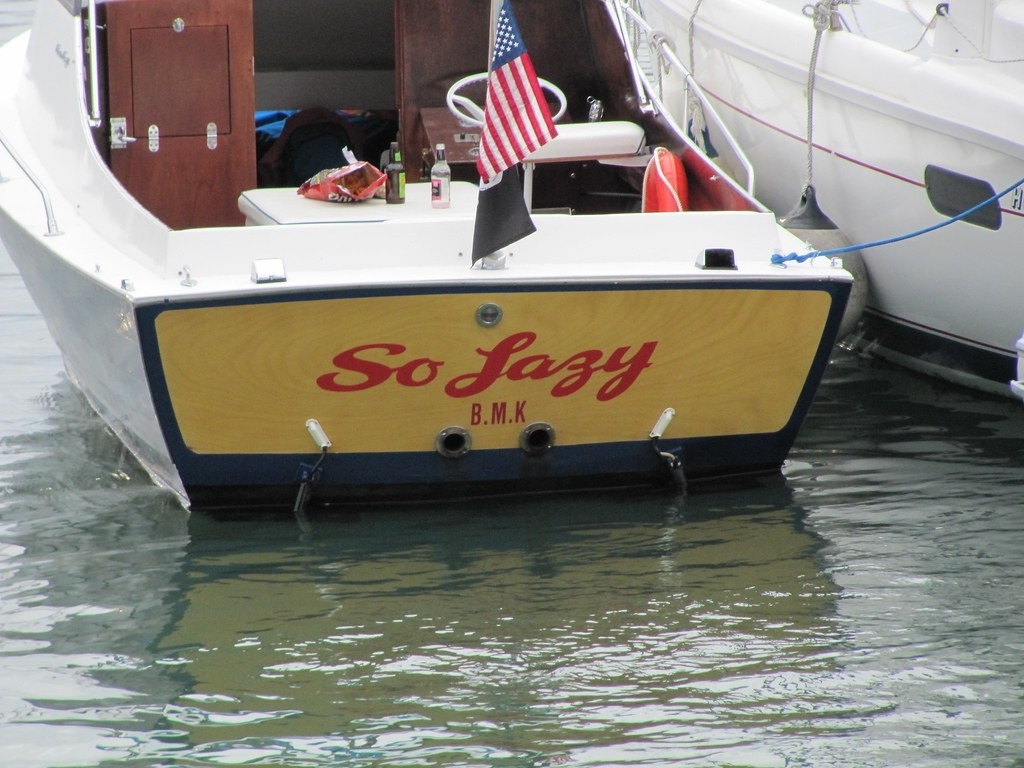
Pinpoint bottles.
[431,144,450,208]
[587,96,604,122]
[384,142,405,204]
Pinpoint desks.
[237,181,480,227]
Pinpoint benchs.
[520,121,646,215]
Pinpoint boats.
[605,0,1024,399]
[0,1,854,514]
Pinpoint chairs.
[261,107,367,187]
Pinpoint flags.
[471,0,559,268]
